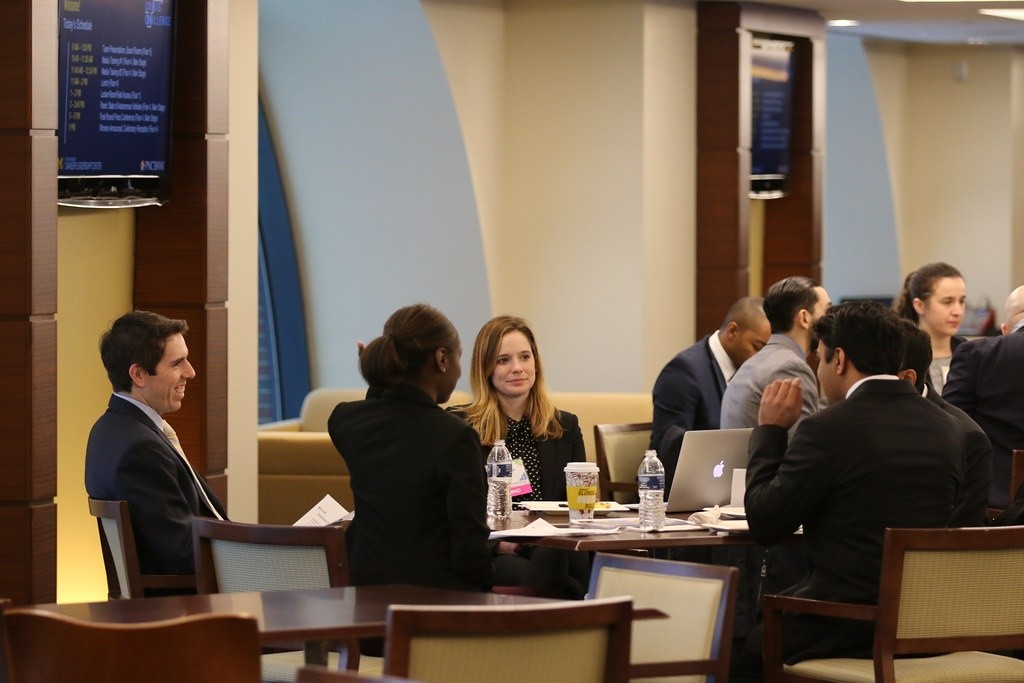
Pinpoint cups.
[563,461,600,523]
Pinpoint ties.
[162,421,223,520]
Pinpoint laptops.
[624,428,755,513]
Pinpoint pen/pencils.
[559,504,568,507]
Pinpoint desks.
[484,510,753,581]
[4,583,669,674]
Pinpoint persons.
[730,301,967,683]
[327,303,503,655]
[86,310,231,598]
[897,262,969,397]
[445,315,593,600]
[901,318,993,527]
[942,287,1024,510]
[720,277,831,450]
[649,298,771,501]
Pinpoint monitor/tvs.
[57,0,180,210]
[747,35,799,199]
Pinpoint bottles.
[637,450,665,531]
[484,439,513,530]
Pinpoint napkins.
[688,503,722,529]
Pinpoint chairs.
[0,497,740,683]
[761,527,1024,683]
[592,420,655,502]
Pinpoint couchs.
[258,386,656,524]
